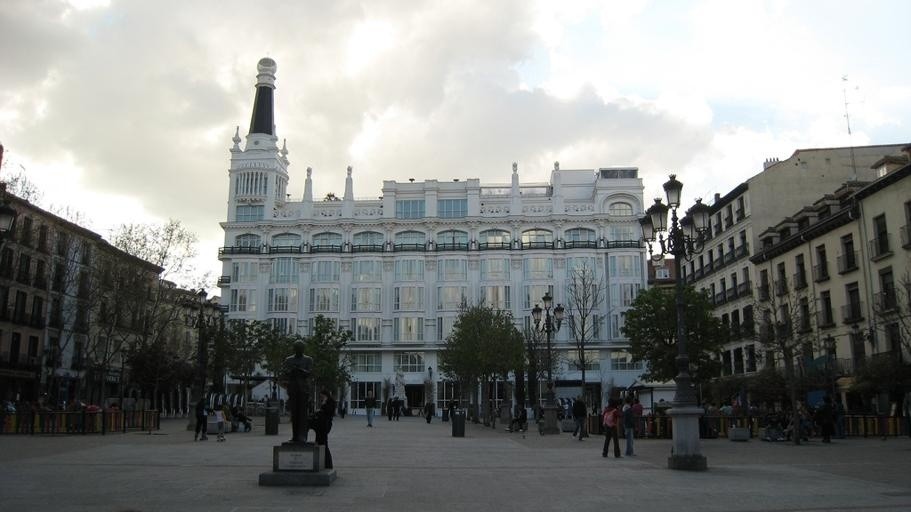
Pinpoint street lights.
[532,291,565,435]
[237,339,255,416]
[638,174,712,472]
[824,333,837,400]
[182,288,222,431]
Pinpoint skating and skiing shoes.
[194,434,226,442]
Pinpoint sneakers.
[764,436,832,444]
[504,425,526,432]
[571,435,592,442]
[603,452,639,459]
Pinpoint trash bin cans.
[452,410,466,436]
[442,407,450,421]
[265,407,279,435]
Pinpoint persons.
[309,390,336,468]
[505,406,527,432]
[364,391,435,428]
[339,396,348,419]
[281,340,314,441]
[571,394,835,458]
[194,397,252,440]
